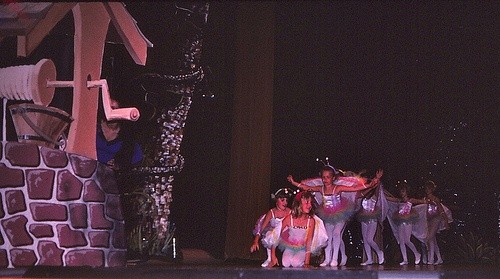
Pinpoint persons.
[249,158,453,269]
[96,98,144,166]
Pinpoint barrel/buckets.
[6,104,75,149]
